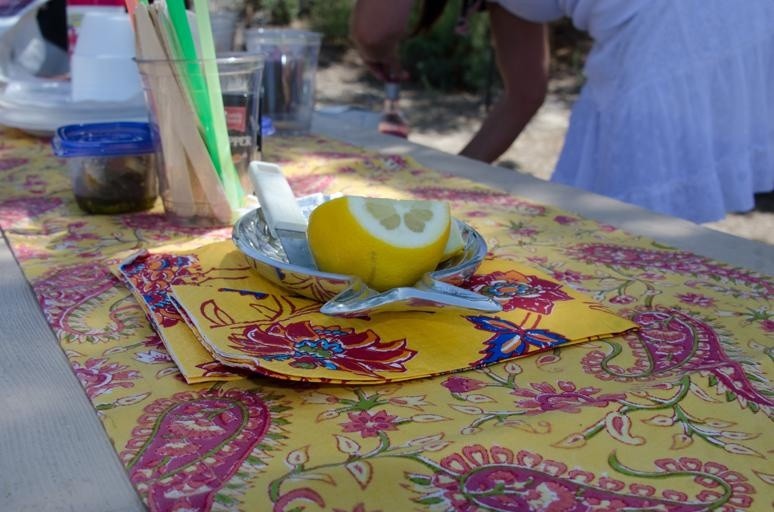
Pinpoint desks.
[0,116,772,512]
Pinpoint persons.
[348,0,772,224]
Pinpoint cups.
[132,51,266,229]
[246,27,324,139]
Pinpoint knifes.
[247,160,319,271]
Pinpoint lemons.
[440,216,466,262]
[308,196,452,291]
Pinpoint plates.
[229,190,505,319]
